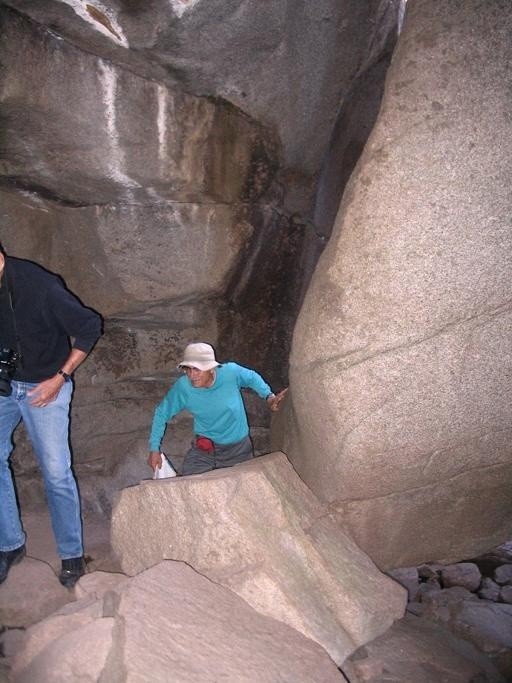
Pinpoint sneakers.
[0,544,26,586]
[59,557,88,586]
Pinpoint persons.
[0,246,102,588]
[146,343,289,477]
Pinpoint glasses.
[181,365,201,372]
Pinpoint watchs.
[57,369,70,382]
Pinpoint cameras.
[0,347,21,397]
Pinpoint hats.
[176,342,220,373]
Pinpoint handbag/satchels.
[195,436,215,453]
